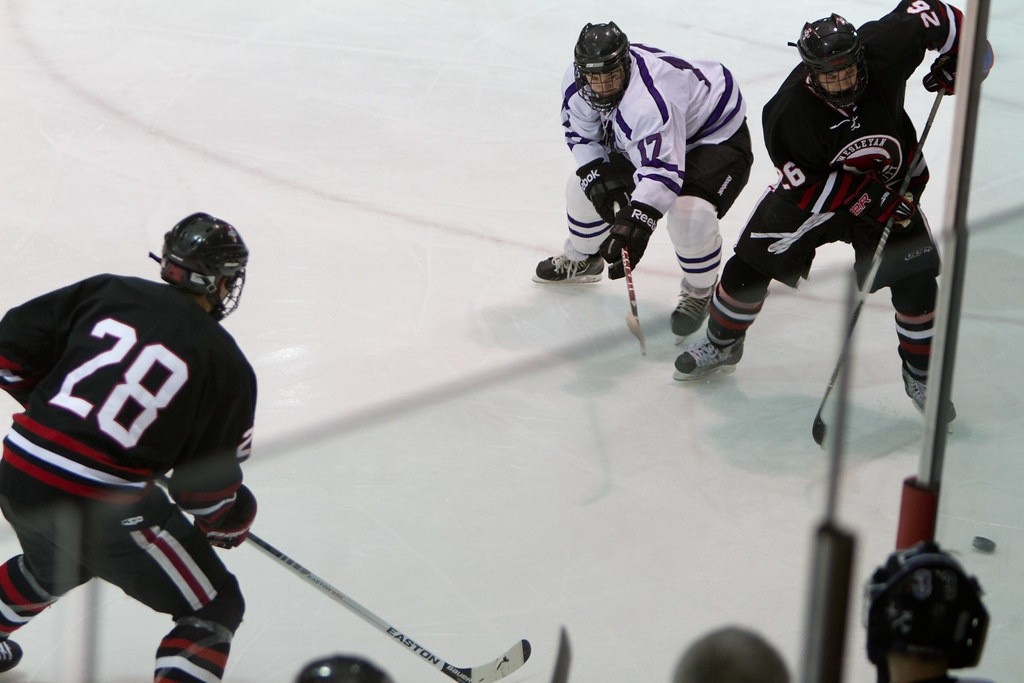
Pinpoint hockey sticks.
[611,200,648,355]
[810,85,947,449]
[551,624,575,683]
[154,471,531,682]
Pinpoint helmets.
[159,213,249,320]
[797,14,869,107]
[862,543,989,670]
[572,21,631,112]
[297,657,383,683]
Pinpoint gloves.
[192,485,259,548]
[845,176,915,231]
[923,41,995,95]
[576,159,631,226]
[599,201,663,280]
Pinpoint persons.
[674,628,790,683]
[530,20,753,345]
[862,539,990,683]
[0,212,258,683]
[672,0,994,434]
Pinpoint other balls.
[972,535,996,555]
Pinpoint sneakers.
[0,639,22,672]
[531,253,603,283]
[672,330,744,381]
[669,283,716,346]
[898,345,956,433]
